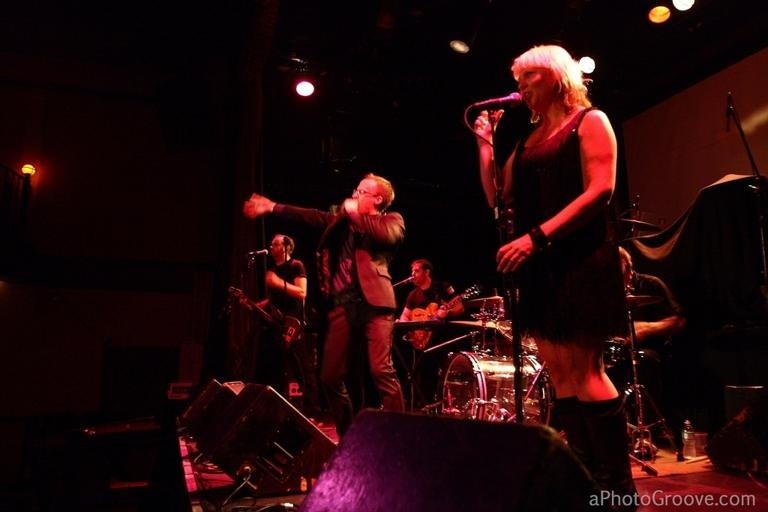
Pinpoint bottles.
[680,418,696,459]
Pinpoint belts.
[326,287,366,308]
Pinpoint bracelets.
[528,225,550,250]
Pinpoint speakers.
[291,407,617,512]
[180,379,338,496]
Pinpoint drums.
[605,340,624,367]
[442,352,551,425]
[495,320,539,356]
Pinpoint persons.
[618,244,686,445]
[472,45,641,512]
[235,235,320,420]
[399,258,466,367]
[241,169,406,438]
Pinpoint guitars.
[406,286,480,350]
[227,286,301,349]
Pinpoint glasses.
[353,189,380,199]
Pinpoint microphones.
[474,91,523,108]
[248,249,268,257]
[724,90,732,134]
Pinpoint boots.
[553,394,640,512]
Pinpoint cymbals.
[448,321,512,330]
[621,218,660,231]
[464,296,503,307]
[625,296,664,309]
